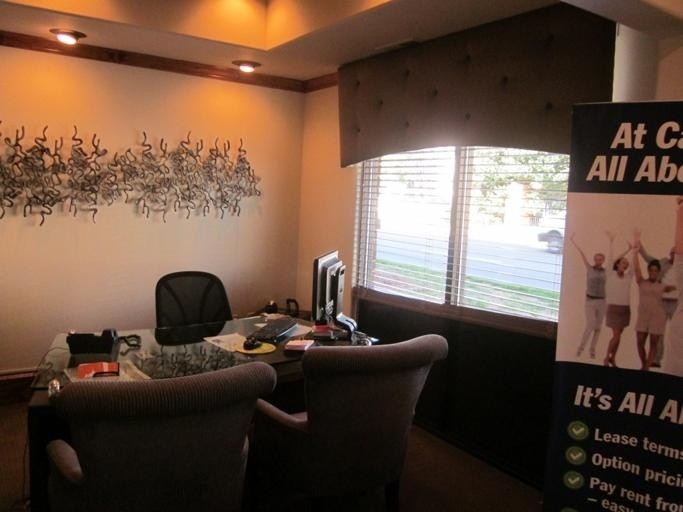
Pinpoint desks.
[30,312,379,391]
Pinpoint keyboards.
[251,318,297,343]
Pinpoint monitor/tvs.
[312,248,358,341]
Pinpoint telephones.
[65,327,119,353]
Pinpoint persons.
[601,237,634,368]
[568,230,606,360]
[631,243,675,372]
[633,229,674,368]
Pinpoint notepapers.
[285,339,315,351]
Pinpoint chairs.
[249,331,447,508]
[43,361,274,508]
[155,270,233,326]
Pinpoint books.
[62,358,151,383]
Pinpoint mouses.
[244,335,261,349]
